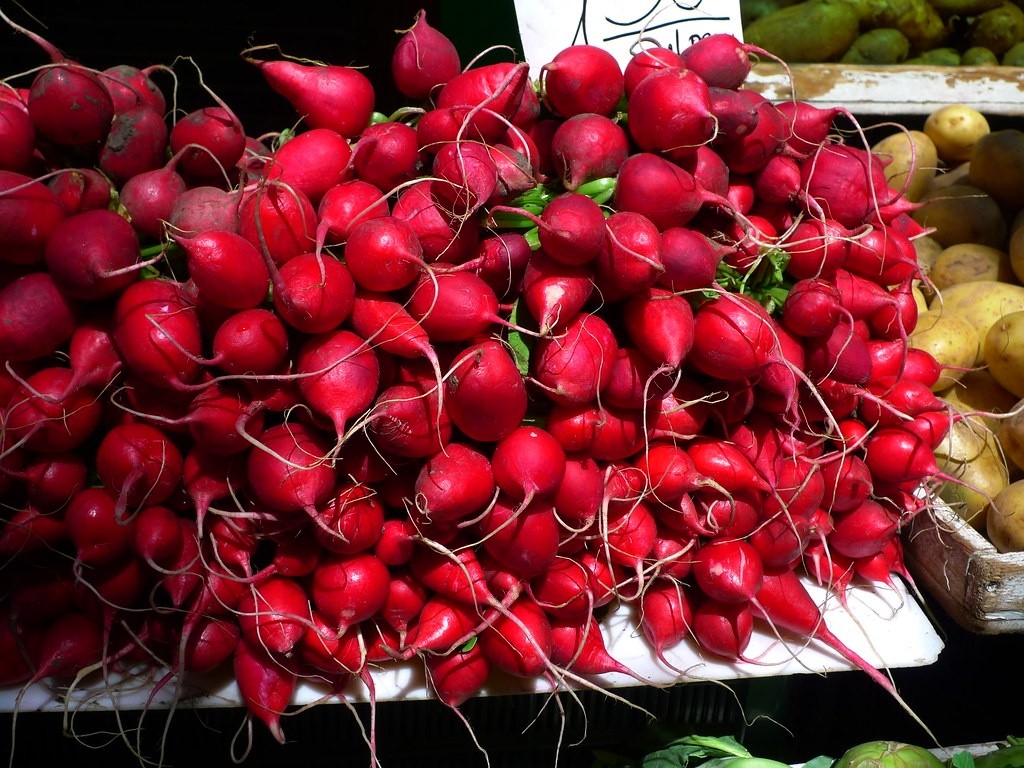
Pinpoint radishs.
[740,0,1024,67]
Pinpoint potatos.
[874,106,1024,553]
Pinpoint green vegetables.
[639,730,754,768]
